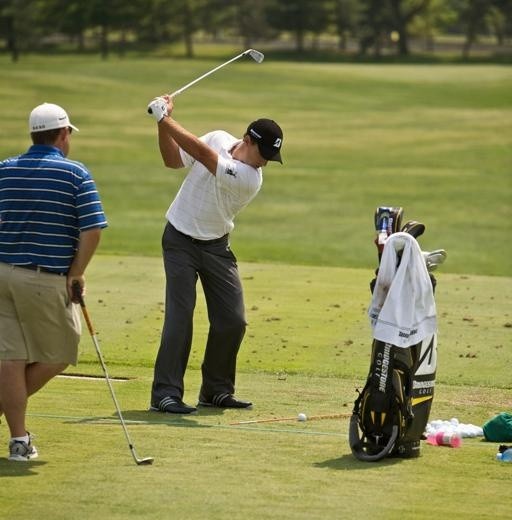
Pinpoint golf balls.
[297,414,306,421]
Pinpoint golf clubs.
[374,206,446,271]
[72,280,153,465]
[148,49,265,114]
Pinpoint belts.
[15,263,68,277]
[168,220,229,245]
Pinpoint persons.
[0,102,108,463]
[147,93,283,416]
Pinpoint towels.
[368,232,438,349]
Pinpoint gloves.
[148,96,169,125]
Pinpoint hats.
[245,118,284,165]
[27,102,81,133]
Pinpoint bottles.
[497,449,512,462]
[424,430,461,447]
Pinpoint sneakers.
[8,431,40,462]
[198,385,253,409]
[149,393,199,415]
